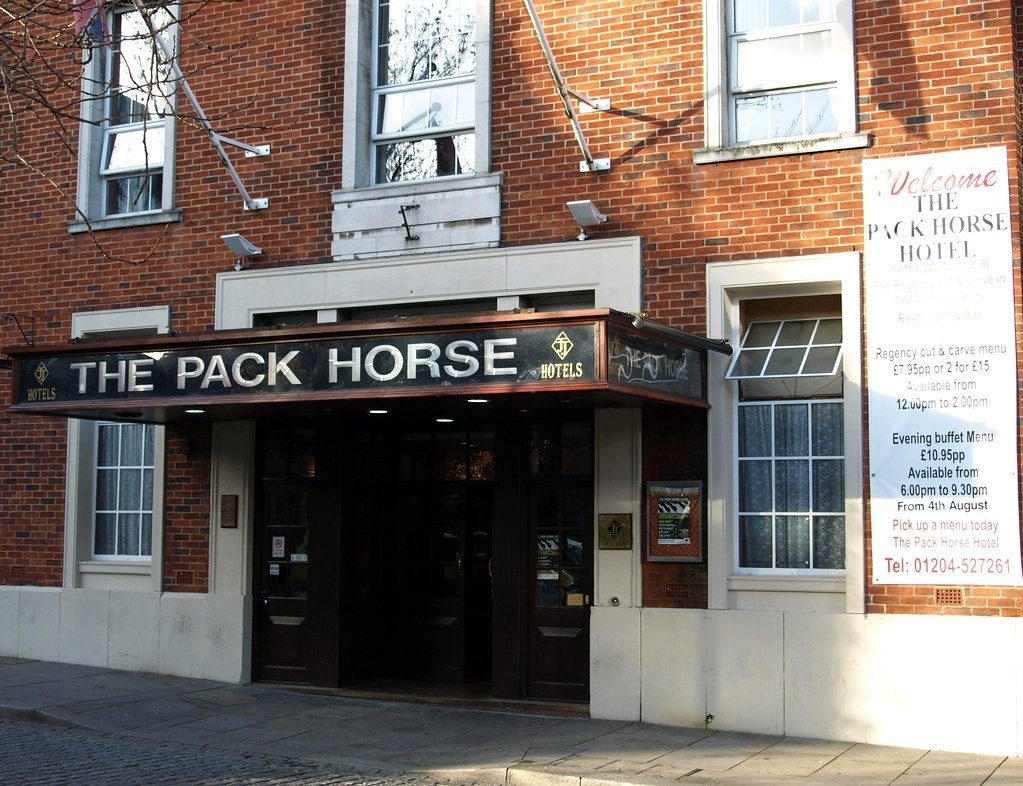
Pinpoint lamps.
[220,233,262,257]
[632,316,644,330]
[566,198,606,227]
